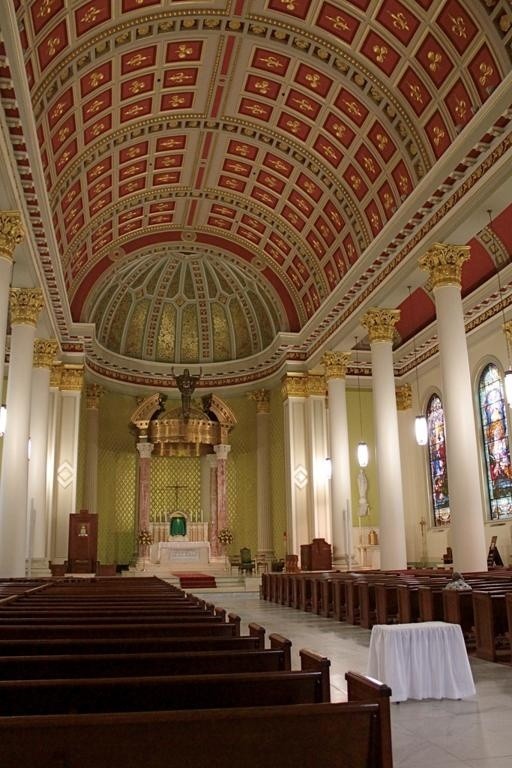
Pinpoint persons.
[166,363,205,423]
[442,569,473,592]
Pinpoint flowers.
[136,532,154,544]
[219,527,232,543]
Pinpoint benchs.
[0,574,396,768]
[259,568,512,667]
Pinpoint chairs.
[239,547,256,577]
[167,510,190,542]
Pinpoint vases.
[220,543,230,556]
[140,545,149,558]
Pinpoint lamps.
[355,336,369,468]
[405,283,432,447]
[324,390,332,482]
[484,208,512,408]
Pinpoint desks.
[149,542,210,565]
[366,619,475,703]
